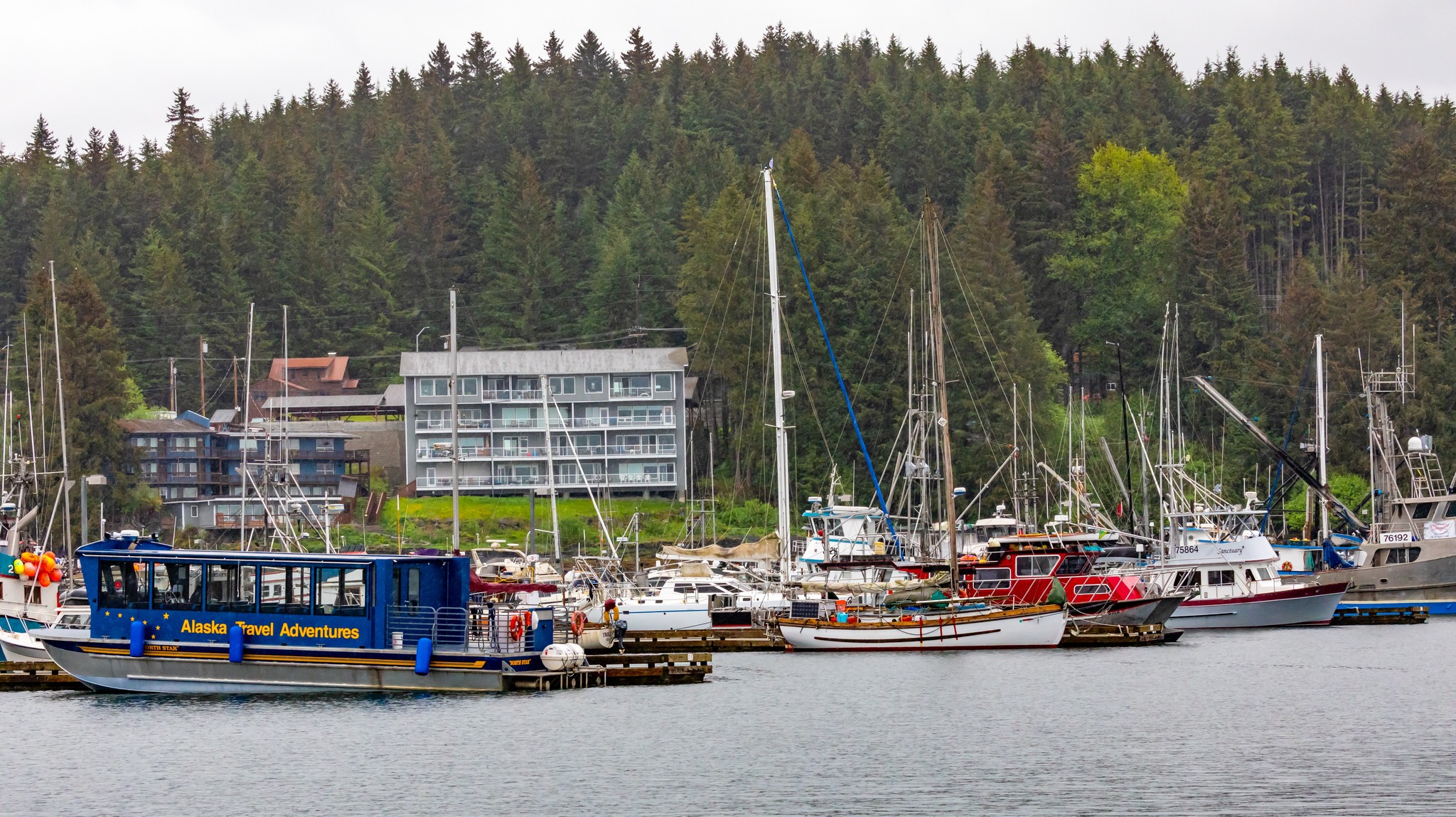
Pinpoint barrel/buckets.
[875,543,884,554]
[837,612,848,622]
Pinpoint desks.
[518,422,524,427]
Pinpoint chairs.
[621,475,626,483]
[628,475,634,483]
[511,420,516,427]
[524,420,531,428]
[645,475,650,482]
[636,475,642,482]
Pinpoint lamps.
[273,472,279,482]
[281,472,289,482]
[151,532,160,539]
[104,532,111,539]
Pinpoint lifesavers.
[570,611,585,636]
[1282,561,1292,571]
[511,615,523,641]
[1368,526,1373,537]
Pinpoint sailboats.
[0,164,1456,702]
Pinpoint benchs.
[128,600,365,617]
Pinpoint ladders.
[1383,494,1390,530]
